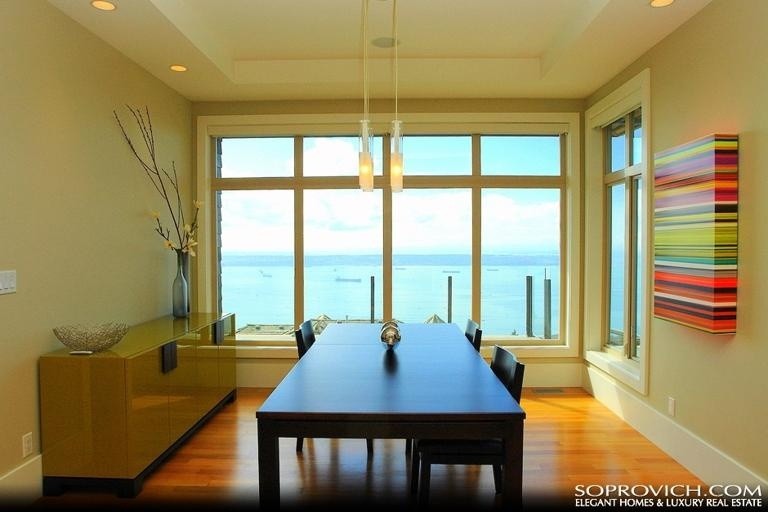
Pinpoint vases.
[172,248,190,317]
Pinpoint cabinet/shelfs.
[40,311,238,499]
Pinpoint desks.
[255,323,527,512]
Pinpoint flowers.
[111,103,202,257]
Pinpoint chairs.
[411,344,525,512]
[406,319,482,460]
[294,320,375,456]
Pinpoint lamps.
[360,2,374,193]
[389,1,404,193]
